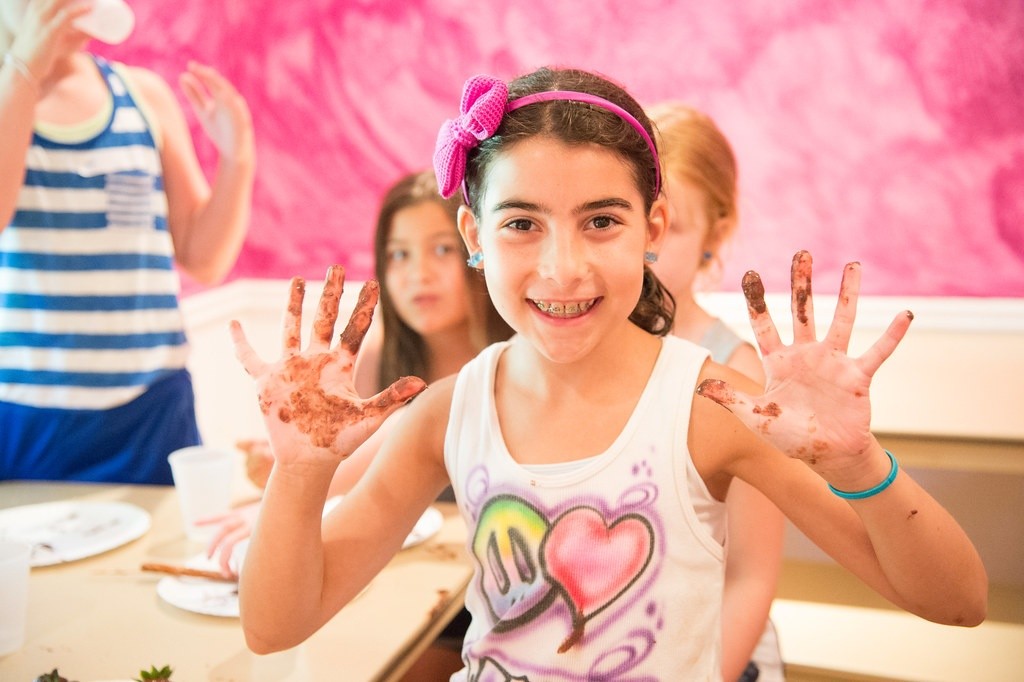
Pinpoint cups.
[168,442,252,544]
[1,543,32,655]
[70,0,136,45]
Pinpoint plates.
[322,494,446,549]
[1,499,152,568]
[155,541,252,617]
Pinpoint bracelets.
[7,54,43,93]
[827,449,899,500]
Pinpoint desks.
[703,295,1024,623]
[769,599,1023,682]
[0,482,474,682]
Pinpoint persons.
[227,65,990,680]
[2,0,259,483]
